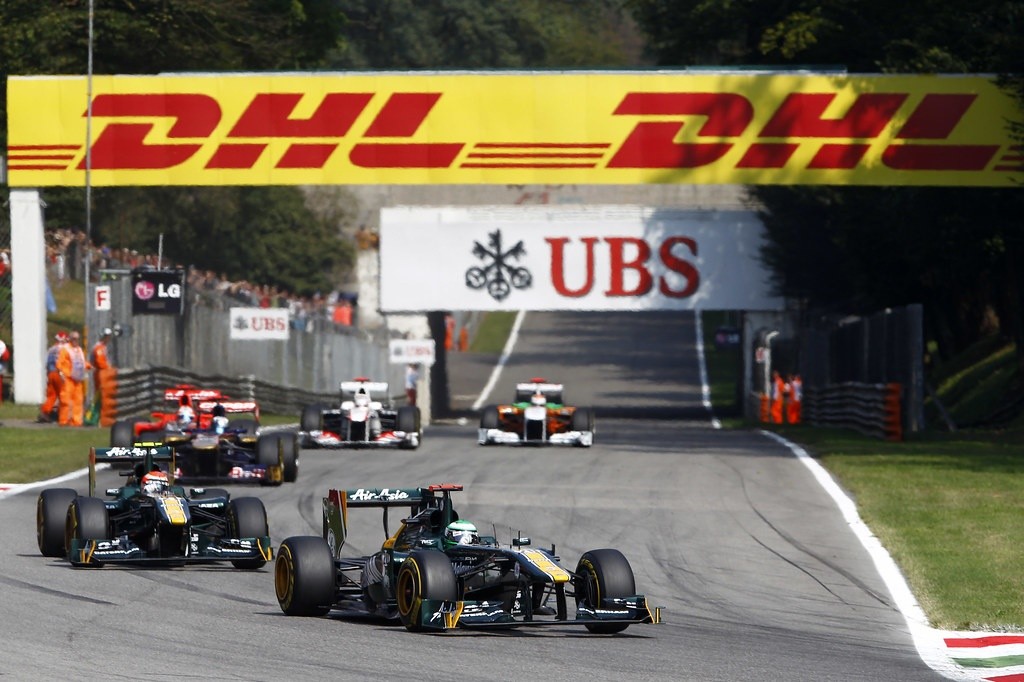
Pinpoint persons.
[139,470,171,499]
[353,386,371,407]
[176,406,198,430]
[405,361,421,406]
[762,371,802,424]
[0,225,359,329]
[0,329,119,427]
[531,392,547,408]
[445,311,455,350]
[460,326,470,351]
[445,520,478,549]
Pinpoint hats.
[55,331,69,342]
[100,328,112,337]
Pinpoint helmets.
[354,386,371,407]
[141,471,170,494]
[443,520,480,550]
[531,390,546,405]
[177,405,194,424]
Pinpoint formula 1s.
[95,385,301,487]
[35,441,276,568]
[477,376,597,448]
[272,481,668,634]
[298,376,423,450]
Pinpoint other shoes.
[38,414,52,423]
